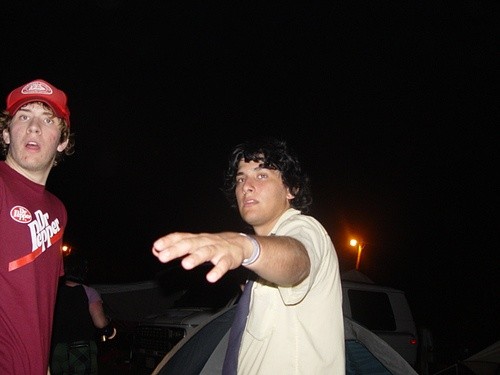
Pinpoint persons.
[149,132,348,375]
[0,80,72,375]
[60,252,124,370]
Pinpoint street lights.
[348,237,364,271]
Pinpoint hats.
[7,78,71,128]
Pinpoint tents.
[150,298,422,375]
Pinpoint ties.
[223,275,256,375]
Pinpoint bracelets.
[240,232,260,266]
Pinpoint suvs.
[133,280,417,371]
